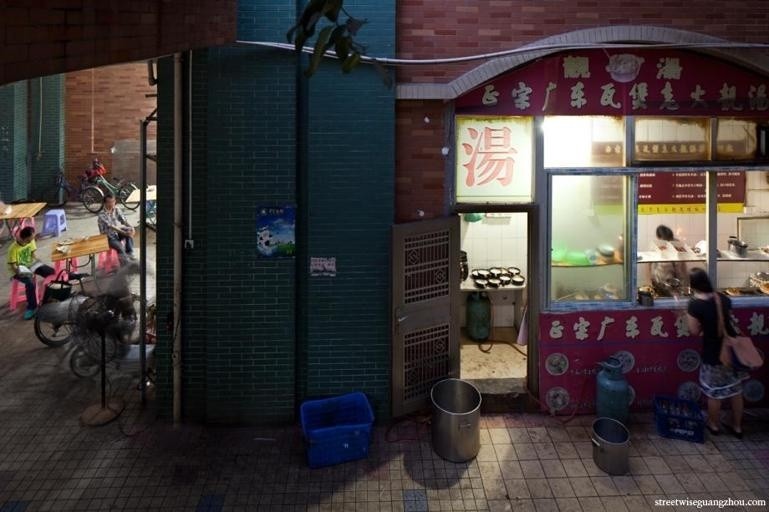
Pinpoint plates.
[743,379,765,403]
[545,351,570,376]
[676,350,705,400]
[545,387,571,411]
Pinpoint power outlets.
[185,240,193,248]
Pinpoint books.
[18,262,44,278]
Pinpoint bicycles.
[42,161,141,213]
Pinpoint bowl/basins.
[471,267,526,289]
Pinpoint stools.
[98,238,126,275]
[41,209,66,237]
[54,256,77,273]
[38,275,67,301]
[10,274,40,311]
[7,217,34,240]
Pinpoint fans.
[68,293,136,427]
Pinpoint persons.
[642,224,688,285]
[682,267,751,438]
[97,194,139,264]
[7,225,56,320]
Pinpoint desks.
[50,233,109,292]
[0,202,48,249]
[124,188,156,232]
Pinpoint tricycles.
[33,273,148,379]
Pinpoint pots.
[727,236,749,258]
[604,54,644,82]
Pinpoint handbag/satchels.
[717,333,764,374]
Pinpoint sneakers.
[22,309,37,320]
[705,420,744,440]
